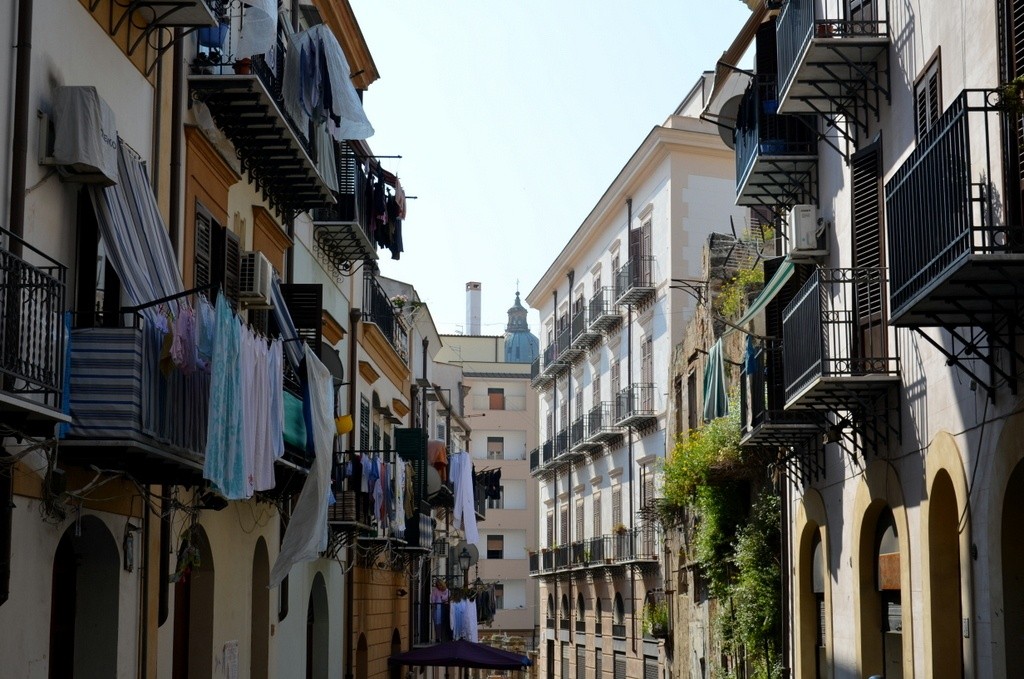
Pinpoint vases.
[233,59,250,75]
[818,25,833,38]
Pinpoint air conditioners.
[781,204,816,256]
[54,85,118,186]
[438,543,448,556]
[237,249,270,306]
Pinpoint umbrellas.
[387,637,529,679]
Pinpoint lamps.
[194,492,228,510]
[432,548,471,579]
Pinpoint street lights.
[458,547,473,679]
